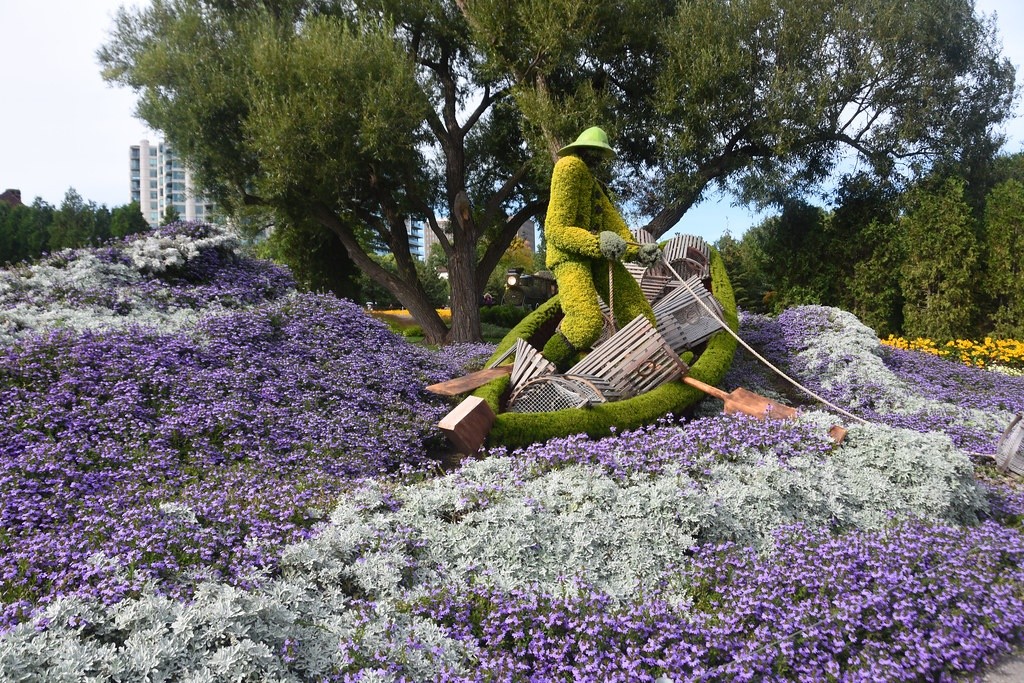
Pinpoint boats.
[473,235,739,453]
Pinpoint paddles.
[682,374,846,452]
[424,361,514,397]
